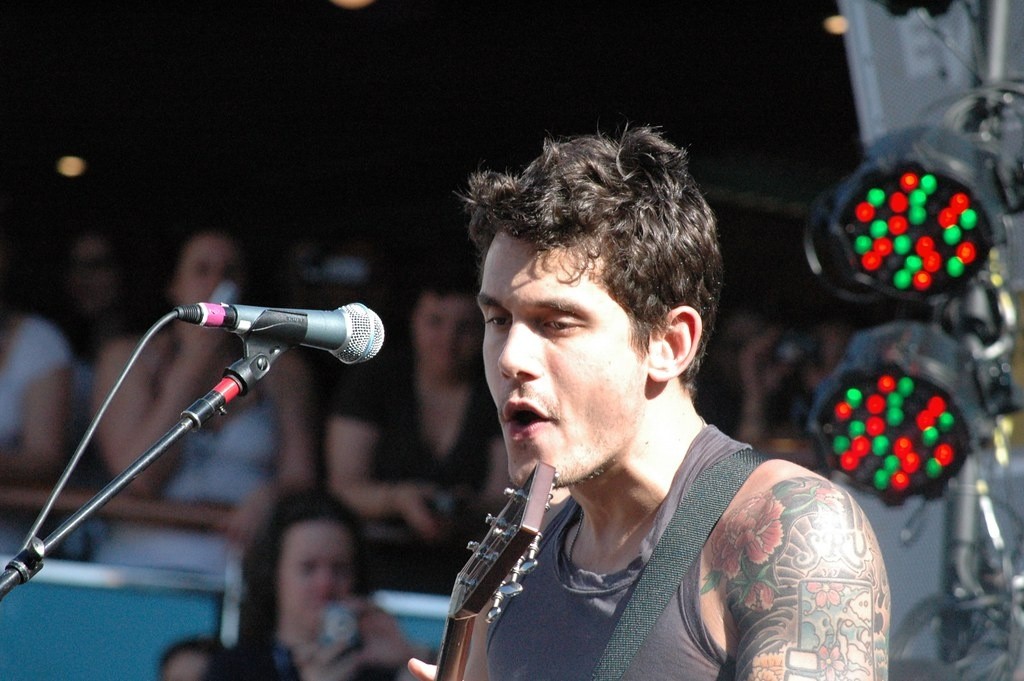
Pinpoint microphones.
[174,302,385,364]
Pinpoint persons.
[409,131,892,681]
[0,231,531,681]
[738,321,850,469]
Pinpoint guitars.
[433,458,560,680]
